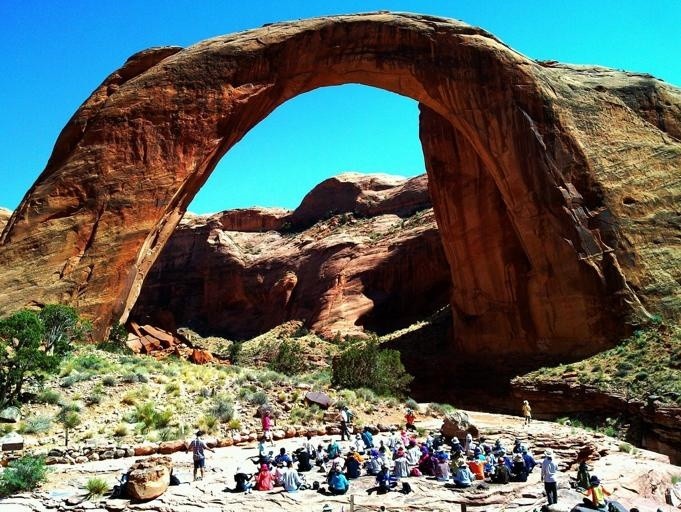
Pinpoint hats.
[544,448,554,458]
[579,462,588,469]
[499,439,527,465]
[458,459,468,466]
[451,434,492,456]
[590,475,600,484]
[524,400,529,404]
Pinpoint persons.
[186,431,216,481]
[234,407,639,512]
[522,401,532,427]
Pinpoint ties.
[436,444,451,452]
